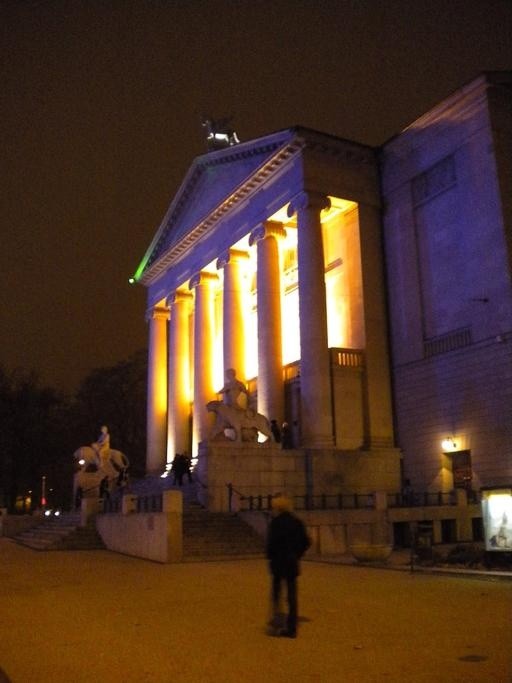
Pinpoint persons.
[266,496,311,638]
[216,368,249,409]
[75,486,84,506]
[271,419,281,443]
[281,422,292,449]
[172,454,192,486]
[117,468,127,485]
[96,426,111,470]
[100,476,109,497]
